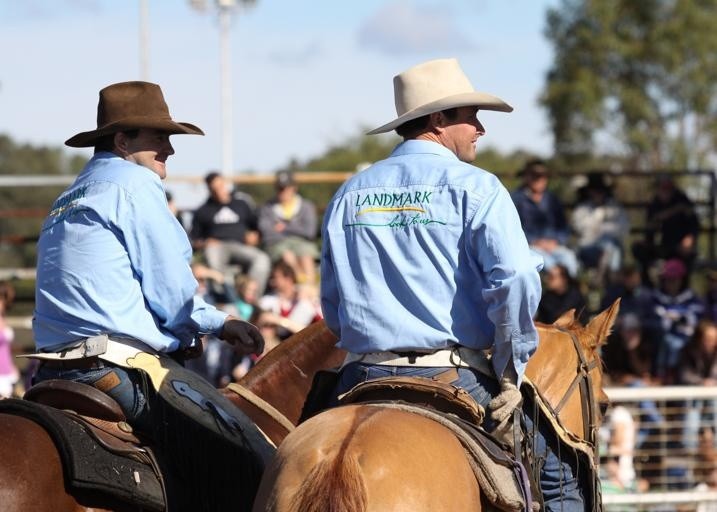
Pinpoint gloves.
[491,361,524,442]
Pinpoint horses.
[250,296,622,512]
[0,318,347,512]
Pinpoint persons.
[2,279,24,401]
[305,59,590,512]
[499,156,717,512]
[157,162,324,378]
[25,79,266,512]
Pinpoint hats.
[65,81,206,147]
[366,58,513,135]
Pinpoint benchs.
[0,228,716,495]
